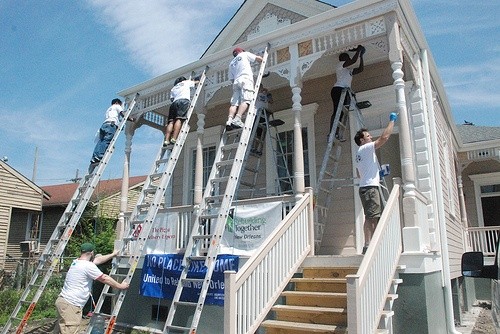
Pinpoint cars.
[461,233,500,333]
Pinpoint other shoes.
[163,138,177,146]
[90,156,100,163]
[225,119,244,130]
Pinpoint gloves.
[379,169,386,177]
[389,112,398,122]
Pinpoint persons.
[54,243,129,334]
[251,100,274,155]
[91,97,133,163]
[328,45,365,141]
[226,47,262,130]
[353,112,399,255]
[164,76,200,145]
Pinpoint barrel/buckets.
[380,164,391,175]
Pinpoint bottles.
[90,313,105,334]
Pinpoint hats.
[232,47,244,56]
[79,243,96,252]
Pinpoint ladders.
[83,65,209,334]
[314,87,390,253]
[232,107,293,200]
[163,41,272,334]
[0,89,140,334]
